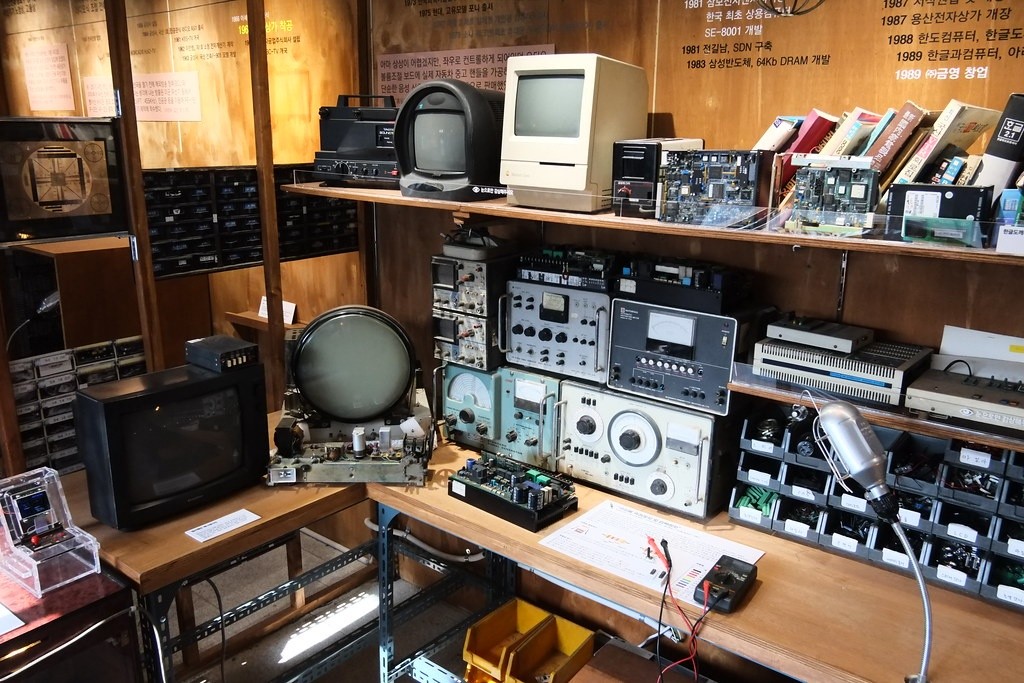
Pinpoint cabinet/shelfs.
[0,525,144,682]
[54,0,1024,681]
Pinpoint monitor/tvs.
[76,361,269,531]
[499,51,649,212]
[392,80,504,201]
